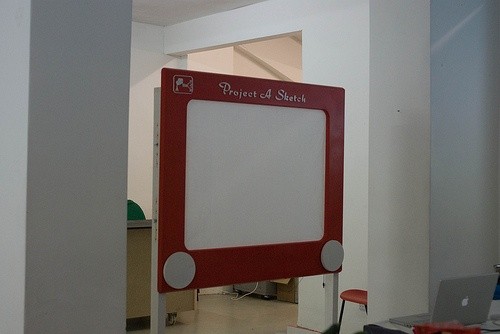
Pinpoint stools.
[336,289,367,334]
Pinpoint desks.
[365,297,499,333]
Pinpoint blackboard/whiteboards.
[157,68,344,294]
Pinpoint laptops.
[388,272,500,329]
